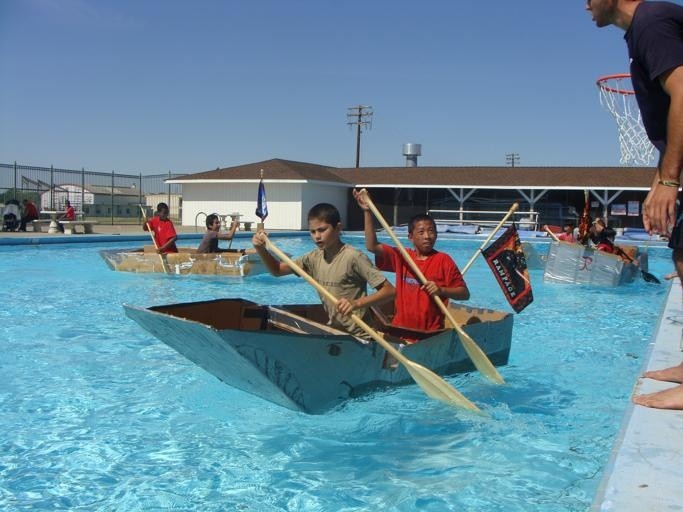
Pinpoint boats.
[95,245,293,278]
[122,297,510,417]
[542,242,648,289]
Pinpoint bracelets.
[656,178,679,189]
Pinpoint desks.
[220,214,244,230]
[39,211,85,233]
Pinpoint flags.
[482,223,533,314]
[254,178,267,221]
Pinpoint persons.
[15,197,39,233]
[663,271,678,280]
[54,199,76,232]
[194,214,238,253]
[142,202,177,254]
[540,214,665,289]
[584,1,682,411]
[351,186,470,345]
[251,202,397,342]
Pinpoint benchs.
[32,219,63,232]
[231,221,255,231]
[58,221,98,234]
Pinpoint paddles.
[604,237,660,284]
[138,202,170,273]
[358,189,506,385]
[259,231,482,414]
